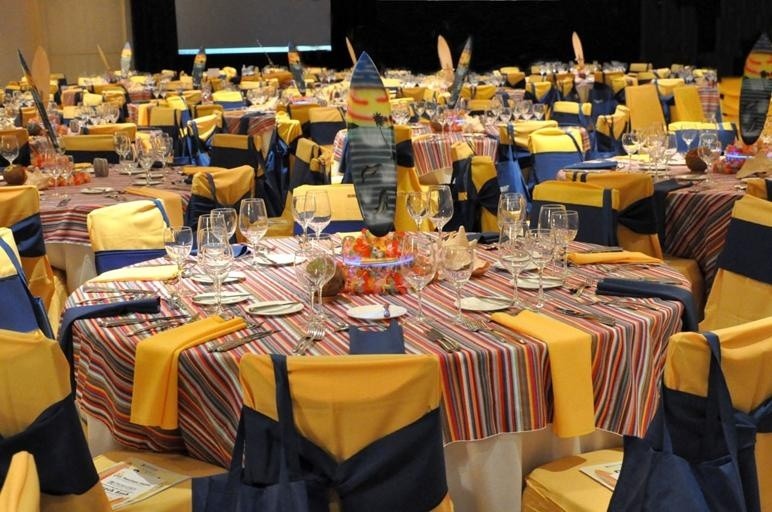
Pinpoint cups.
[138,58,609,108]
[0,90,120,136]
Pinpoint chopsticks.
[98,311,190,331]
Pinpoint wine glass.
[442,244,472,325]
[290,188,333,243]
[162,197,270,322]
[622,120,723,189]
[0,134,20,167]
[113,128,175,188]
[399,232,438,323]
[391,94,547,141]
[292,233,336,322]
[498,192,580,310]
[43,147,74,200]
[405,184,454,235]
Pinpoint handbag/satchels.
[432,156,478,232]
[598,430,749,512]
[187,469,310,512]
[174,121,288,217]
[495,158,531,207]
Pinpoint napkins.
[346,315,406,357]
[594,277,700,337]
[129,314,246,428]
[61,293,159,400]
[487,310,596,439]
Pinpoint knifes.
[81,284,154,294]
[554,306,616,327]
[424,327,460,353]
[207,329,282,354]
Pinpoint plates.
[79,186,113,195]
[244,298,305,318]
[453,292,517,314]
[346,303,407,322]
[255,252,307,267]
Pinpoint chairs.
[2,61,768,314]
[0,329,225,511]
[521,316,768,511]
[191,347,455,509]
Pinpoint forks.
[576,278,592,297]
[463,320,529,346]
[292,323,326,357]
